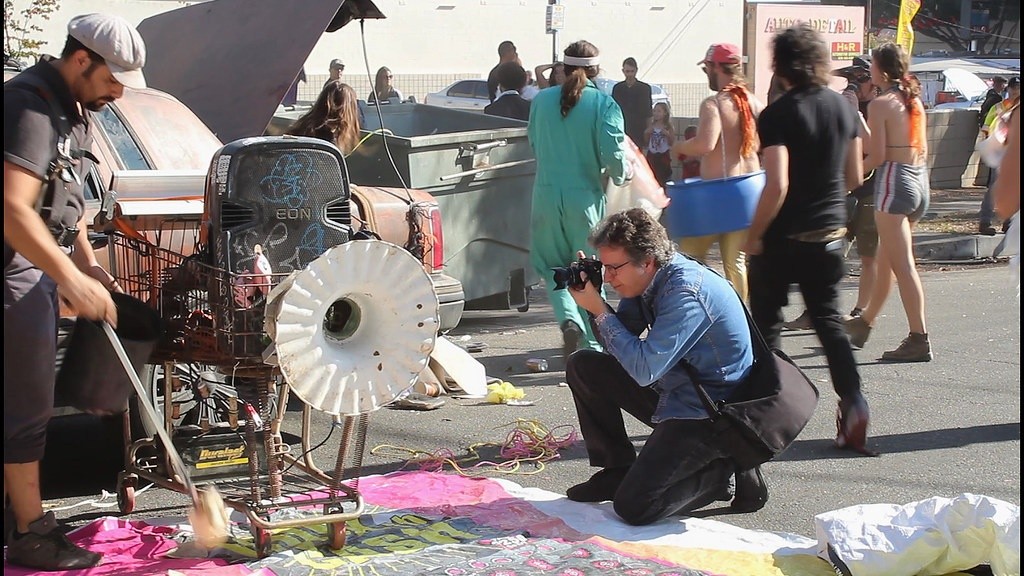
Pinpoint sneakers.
[849,308,861,317]
[784,310,815,330]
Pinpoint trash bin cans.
[273,100,541,313]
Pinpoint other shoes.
[1002,218,1011,233]
[6,511,100,569]
[562,320,583,356]
[566,466,635,502]
[731,464,769,514]
[978,223,996,235]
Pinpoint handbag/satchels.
[711,346,819,460]
[976,134,1009,170]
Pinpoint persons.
[3,10,147,570]
[567,207,771,526]
[285,59,377,162]
[671,20,933,448]
[979,74,1020,280]
[484,39,565,122]
[612,56,677,184]
[367,67,399,103]
[528,40,633,360]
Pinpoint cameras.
[551,258,604,294]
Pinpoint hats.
[696,43,741,64]
[69,11,148,90]
[1008,77,1020,87]
[994,77,1007,83]
[330,59,344,69]
[832,54,872,77]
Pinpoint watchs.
[595,309,612,324]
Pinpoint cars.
[0,0,467,375]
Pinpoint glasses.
[847,73,871,83]
[604,261,630,276]
[384,75,393,79]
[1010,83,1020,89]
[333,65,343,70]
[702,67,709,75]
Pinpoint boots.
[842,314,872,348]
[882,332,931,363]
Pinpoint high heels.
[832,393,869,450]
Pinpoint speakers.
[206,132,351,357]
